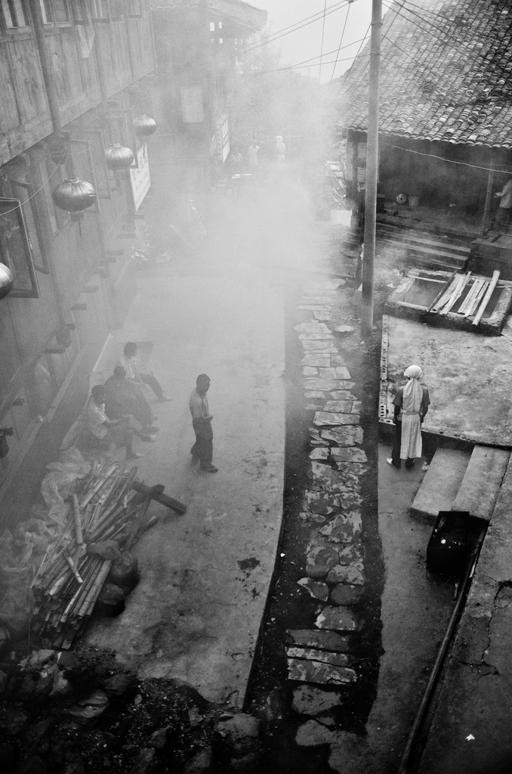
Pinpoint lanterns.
[103,142,134,180]
[53,175,98,236]
[0,262,18,301]
[129,112,156,155]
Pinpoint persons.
[86,384,158,460]
[493,176,511,232]
[272,133,288,161]
[243,140,262,168]
[226,145,244,169]
[104,366,160,434]
[188,372,219,474]
[384,363,431,469]
[116,341,174,403]
